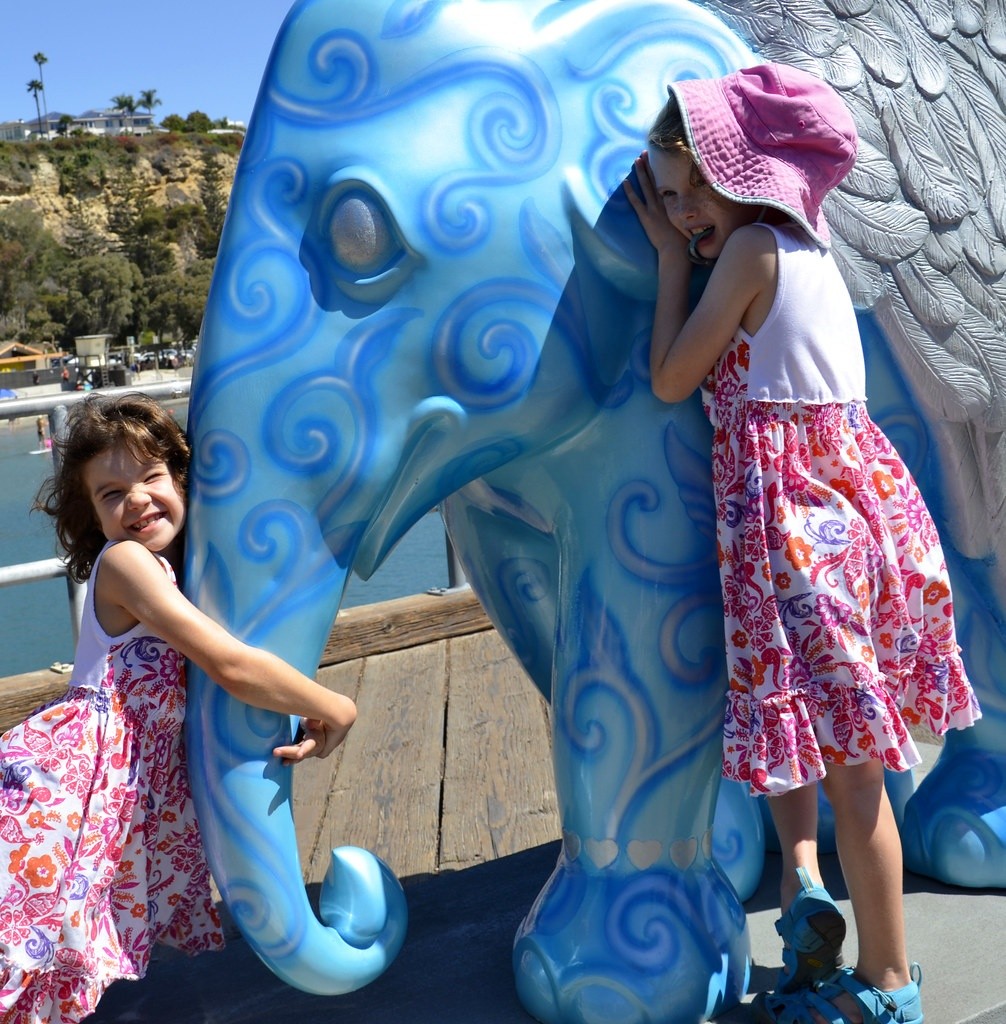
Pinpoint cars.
[0,388,17,401]
[50,340,197,366]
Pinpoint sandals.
[774,885,846,993]
[751,964,924,1024]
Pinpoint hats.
[668,63,858,250]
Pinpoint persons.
[625,60,981,1024]
[0,392,359,1024]
[33,371,39,384]
[61,366,70,382]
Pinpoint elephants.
[189,0,1006,1024]
